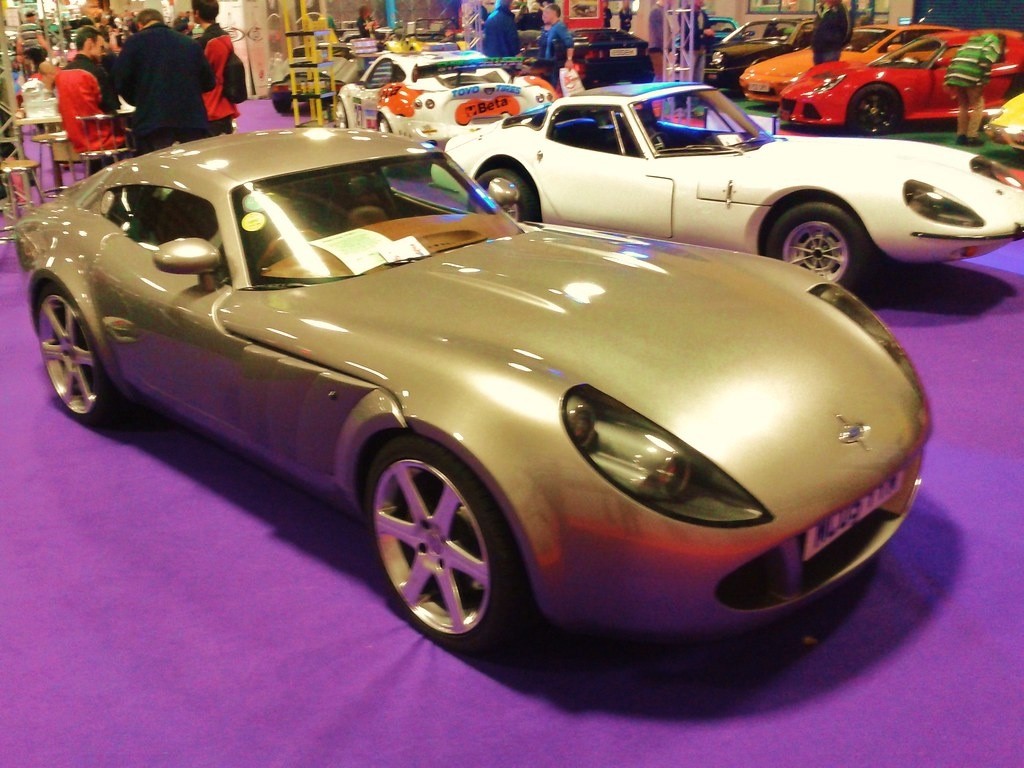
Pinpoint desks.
[11,104,137,210]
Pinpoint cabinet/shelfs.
[282,0,338,128]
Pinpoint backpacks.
[206,32,247,104]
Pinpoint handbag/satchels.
[559,68,586,97]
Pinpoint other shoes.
[966,137,985,146]
[956,135,966,144]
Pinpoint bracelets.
[567,58,572,60]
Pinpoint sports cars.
[432,81,1022,287]
[268,19,541,116]
[778,25,1024,135]
[982,91,1023,151]
[337,52,558,149]
[13,126,936,661]
[740,24,967,104]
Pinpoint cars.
[696,15,855,88]
[515,25,654,89]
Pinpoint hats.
[26,11,35,17]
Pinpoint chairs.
[554,110,660,157]
[155,187,224,246]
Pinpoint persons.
[812,0,852,63]
[619,1,632,31]
[471,0,487,30]
[482,0,522,57]
[602,1,612,27]
[16,13,46,62]
[538,4,574,87]
[515,5,544,30]
[647,0,715,82]
[56,0,240,170]
[356,4,379,37]
[944,32,1007,146]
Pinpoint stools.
[0,112,131,242]
